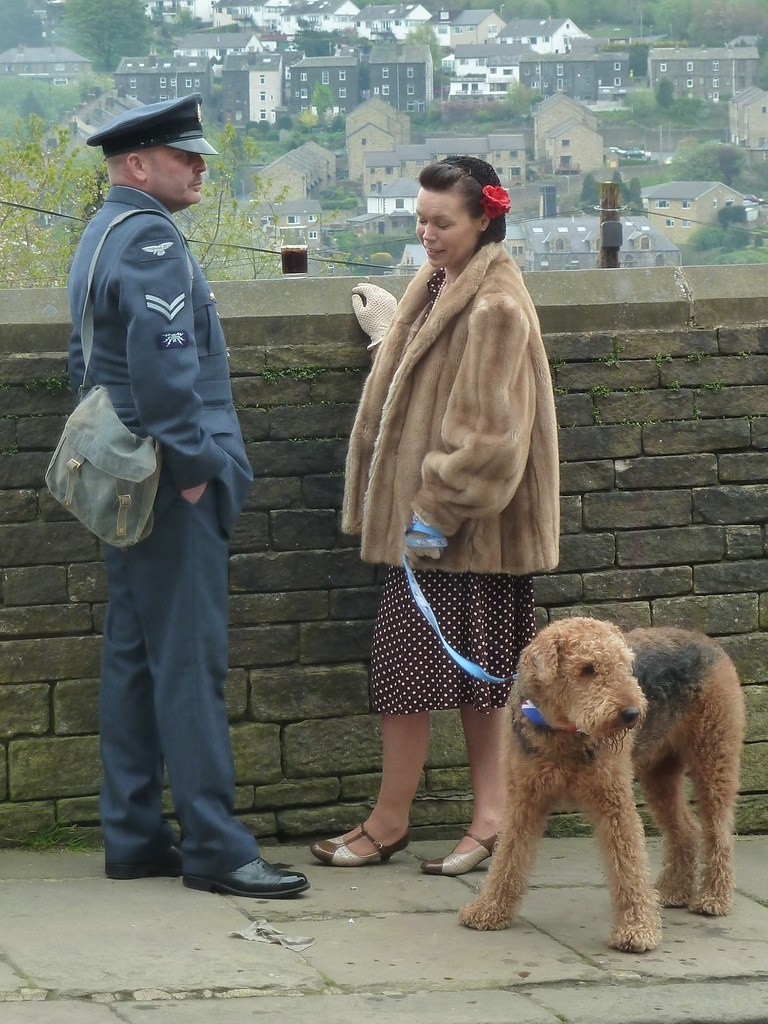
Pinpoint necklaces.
[430,278,447,312]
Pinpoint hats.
[86,91,217,157]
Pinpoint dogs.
[459,616,743,954]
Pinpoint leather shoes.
[104,847,309,897]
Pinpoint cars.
[625,148,651,160]
[609,146,626,155]
[744,194,764,204]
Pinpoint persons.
[306,154,566,880]
[64,90,315,898]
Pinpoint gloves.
[405,515,443,564]
[352,283,398,350]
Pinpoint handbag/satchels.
[45,386,162,551]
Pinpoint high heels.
[310,823,502,875]
